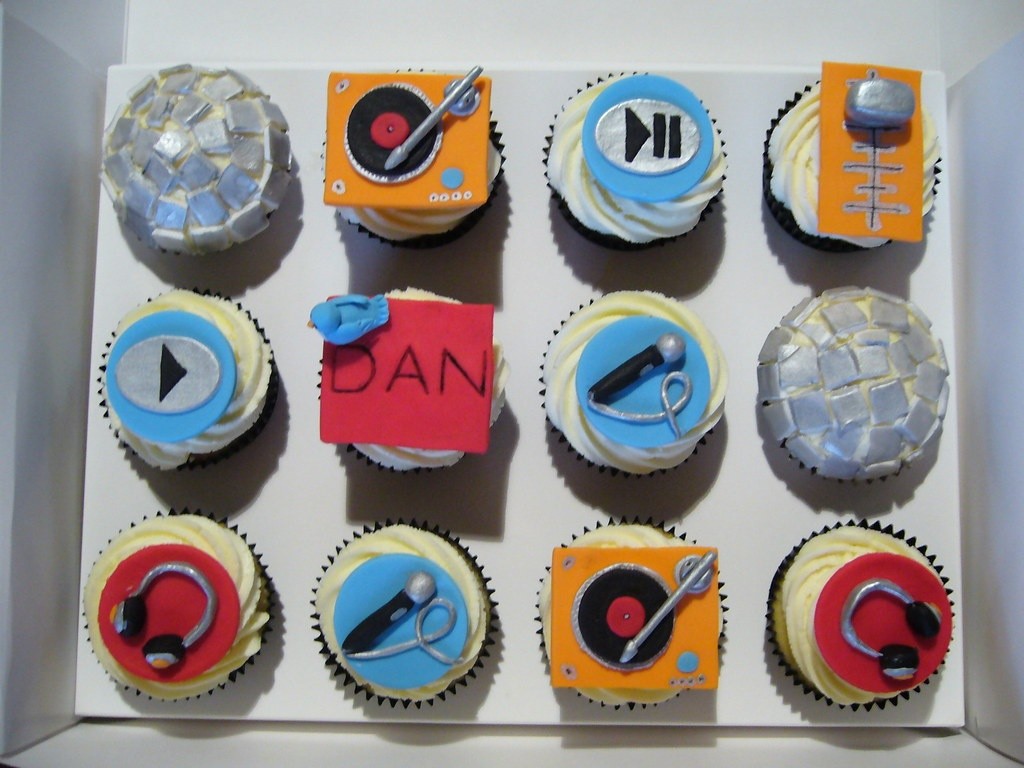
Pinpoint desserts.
[81,63,954,710]
